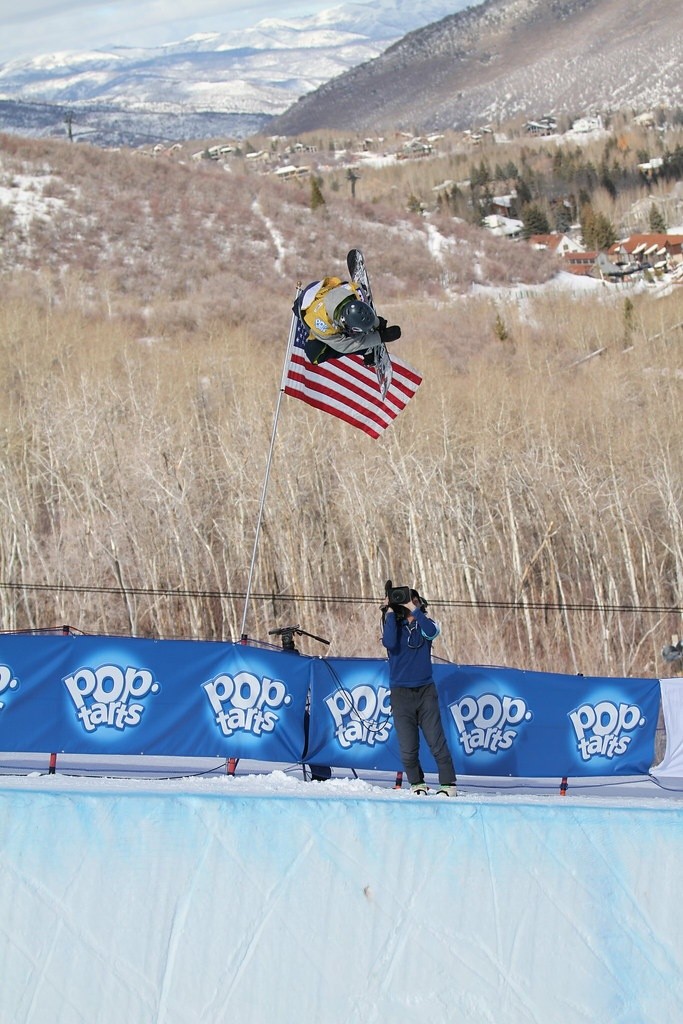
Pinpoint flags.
[283,318,424,440]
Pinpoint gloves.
[380,325,401,343]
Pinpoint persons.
[292,276,401,365]
[380,589,457,798]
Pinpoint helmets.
[340,301,375,333]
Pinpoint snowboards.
[347,249,393,401]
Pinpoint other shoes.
[364,353,374,366]
[437,784,456,797]
[410,782,428,795]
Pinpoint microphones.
[385,580,392,590]
